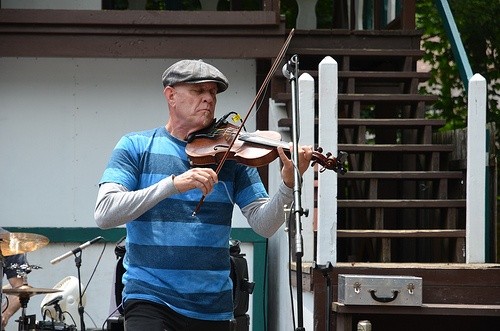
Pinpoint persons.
[0,227,28,331]
[94,59,315,331]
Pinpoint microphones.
[281,55,299,79]
[50,236,103,264]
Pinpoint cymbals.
[1,284,64,298]
[0,233,49,257]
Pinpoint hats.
[161,58,230,94]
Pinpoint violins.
[185,117,348,174]
